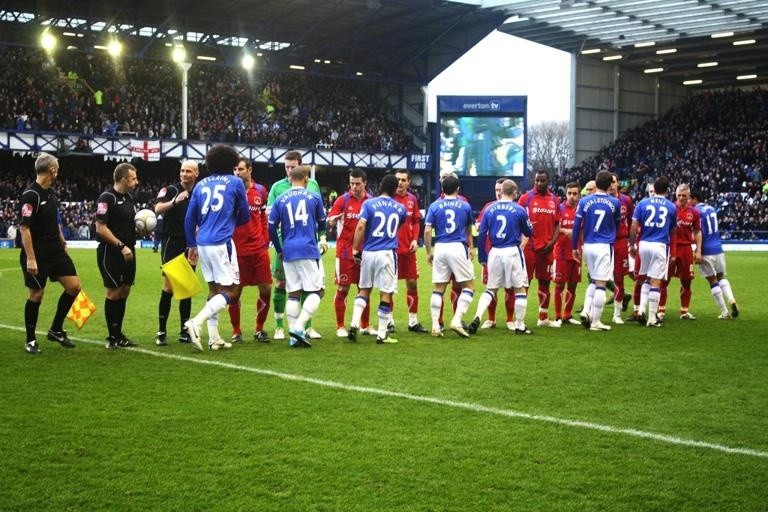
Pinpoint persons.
[14,153,82,353]
[0,42,416,155]
[0,159,171,244]
[549,86,768,241]
[91,162,152,348]
[153,145,739,355]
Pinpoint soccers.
[134,209,158,231]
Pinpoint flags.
[67,289,96,330]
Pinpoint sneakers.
[335,323,399,344]
[731,300,739,318]
[25,340,42,354]
[47,328,76,347]
[681,313,697,321]
[179,319,322,353]
[718,313,730,320]
[537,309,666,331]
[105,335,137,348]
[408,319,497,338]
[156,332,168,345]
[507,321,533,334]
